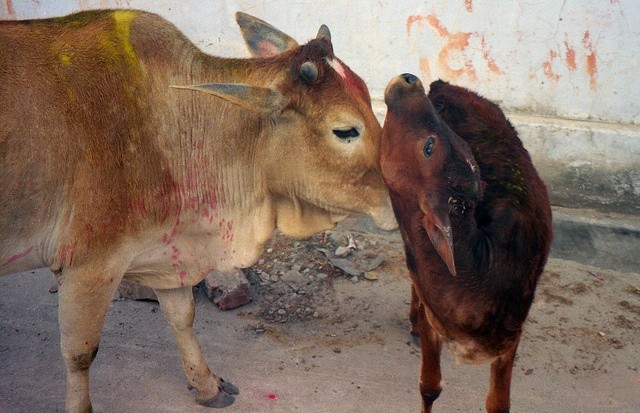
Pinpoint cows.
[0,8,400,413]
[379,73,553,413]
